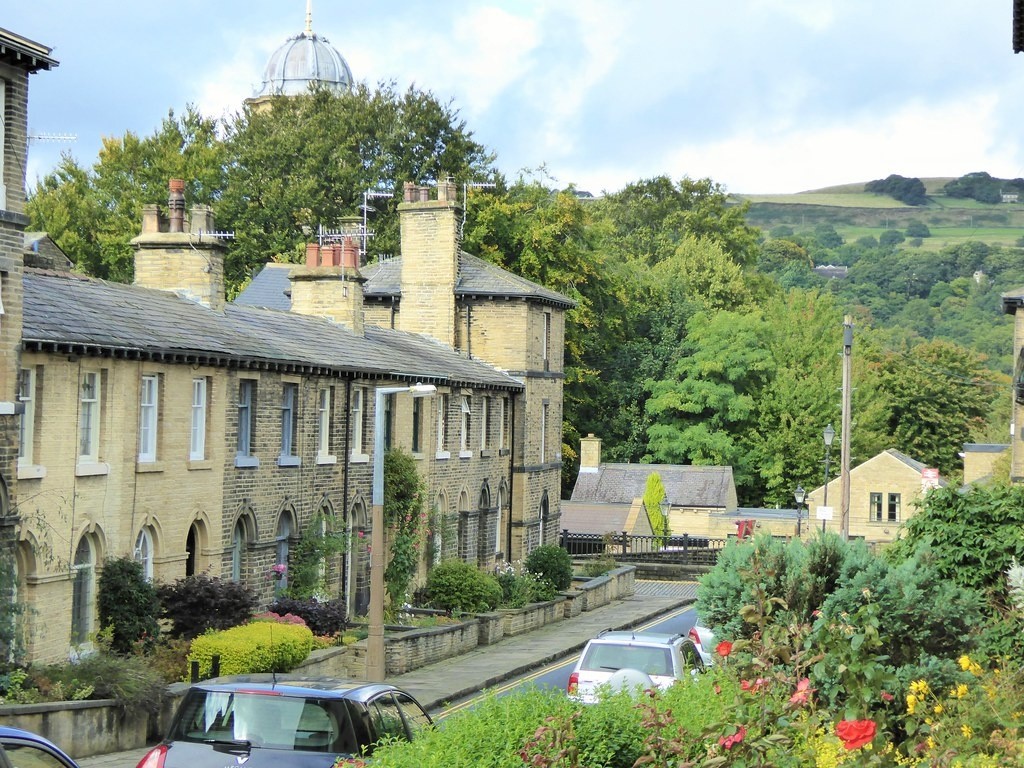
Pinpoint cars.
[687,616,720,667]
[132,672,439,768]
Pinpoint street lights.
[819,423,837,534]
[362,382,438,685]
[792,484,805,539]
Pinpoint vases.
[274,571,282,580]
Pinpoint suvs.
[564,627,707,714]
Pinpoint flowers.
[273,565,286,573]
